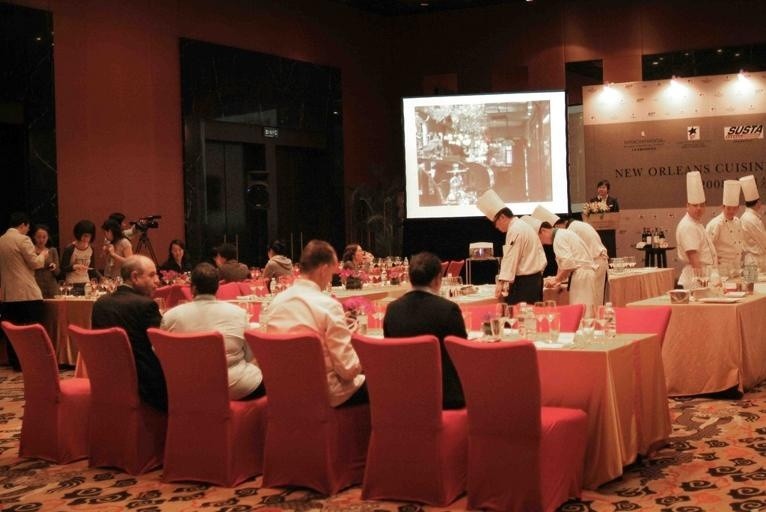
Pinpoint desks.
[40,292,100,368]
[229,280,415,305]
[436,266,677,317]
[626,278,766,398]
[633,235,677,267]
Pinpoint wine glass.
[371,256,401,269]
[368,269,381,287]
[480,301,517,343]
[534,300,608,347]
[609,256,637,276]
[277,275,291,293]
[248,266,265,300]
[369,299,386,336]
[56,276,116,300]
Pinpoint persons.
[265,243,294,278]
[151,262,265,401]
[345,245,364,267]
[93,256,167,406]
[674,171,764,292]
[0,210,145,371]
[476,190,609,322]
[384,251,469,410]
[260,241,369,408]
[591,180,619,258]
[212,244,254,280]
[165,240,192,272]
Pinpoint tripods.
[134,235,158,264]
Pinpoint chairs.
[349,330,467,505]
[247,302,672,344]
[146,327,268,489]
[443,330,592,510]
[67,323,165,478]
[0,319,92,463]
[244,328,361,499]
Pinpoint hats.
[475,189,507,221]
[722,175,759,206]
[686,171,705,205]
[520,204,560,235]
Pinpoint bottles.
[258,302,268,335]
[403,256,408,265]
[357,303,368,335]
[270,277,277,292]
[184,270,192,283]
[518,302,537,343]
[382,268,388,283]
[642,225,665,249]
[603,300,617,340]
